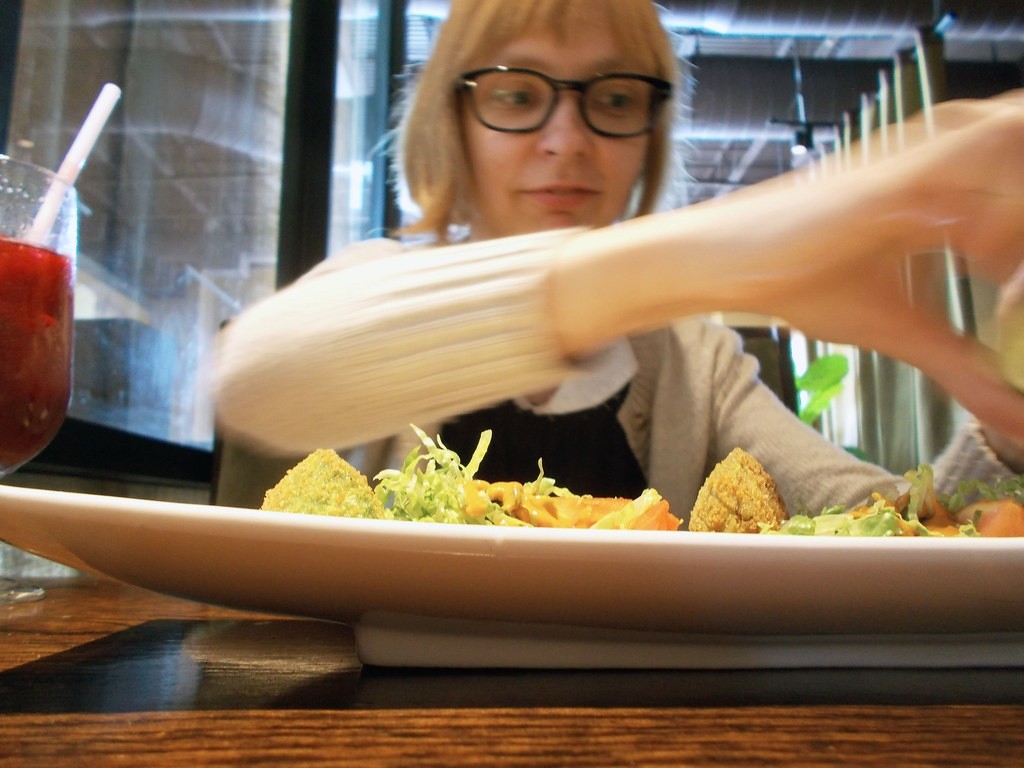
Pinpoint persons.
[199,0,1024,532]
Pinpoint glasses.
[456,65,674,140]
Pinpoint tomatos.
[949,496,1024,537]
[520,496,683,531]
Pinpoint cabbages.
[370,420,662,527]
[758,466,1024,539]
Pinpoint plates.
[0,482,1024,671]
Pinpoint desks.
[0,575,1024,768]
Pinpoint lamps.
[789,123,814,155]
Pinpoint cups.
[0,154,79,605]
